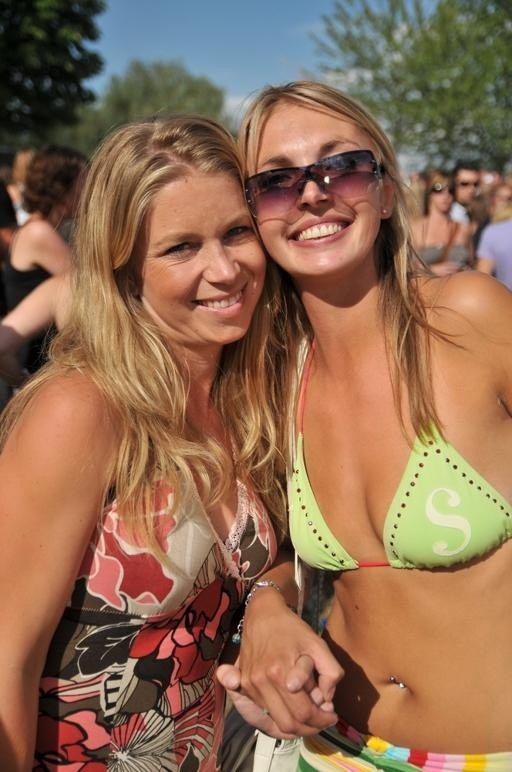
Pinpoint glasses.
[430,183,455,194]
[242,150,387,219]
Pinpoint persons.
[1,145,92,409]
[399,158,511,292]
[235,80,511,772]
[2,114,334,772]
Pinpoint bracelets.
[232,580,280,644]
